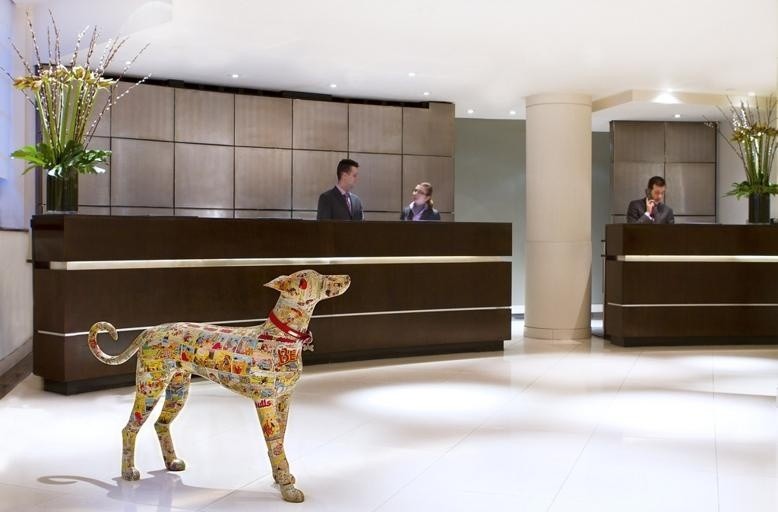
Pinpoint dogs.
[86,266,354,504]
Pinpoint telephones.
[646,189,656,208]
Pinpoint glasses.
[414,189,429,196]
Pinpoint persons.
[627,175,675,224]
[399,182,441,221]
[318,159,365,219]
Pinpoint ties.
[345,192,353,212]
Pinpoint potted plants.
[703,90,778,223]
[0,10,153,214]
